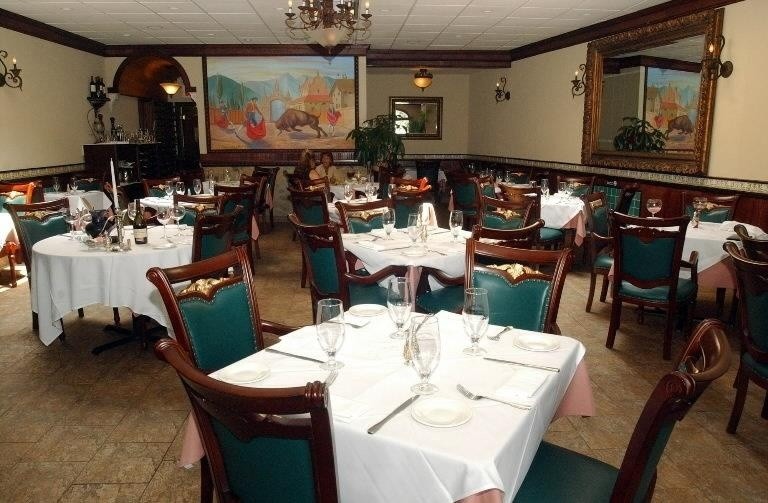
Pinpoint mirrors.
[389,94,443,142]
[579,6,726,180]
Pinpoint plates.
[409,396,472,428]
[217,362,268,384]
[348,302,386,317]
[512,330,559,352]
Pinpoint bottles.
[85,210,114,238]
[133,198,147,244]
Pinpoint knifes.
[365,390,420,435]
[262,347,325,366]
[483,356,559,372]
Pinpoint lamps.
[159,64,185,100]
[412,67,435,90]
[694,26,736,81]
[0,47,28,90]
[572,62,593,99]
[284,0,373,55]
[491,73,513,101]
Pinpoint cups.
[127,202,135,221]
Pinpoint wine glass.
[100,124,156,142]
[48,176,79,197]
[468,163,573,201]
[62,207,91,241]
[407,314,441,397]
[156,206,186,238]
[647,198,663,219]
[382,207,464,247]
[342,165,397,203]
[386,276,412,338]
[163,168,242,200]
[462,286,489,356]
[317,297,345,372]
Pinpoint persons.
[294,151,329,190]
[244,96,263,126]
[316,151,340,184]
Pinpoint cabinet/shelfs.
[82,140,164,182]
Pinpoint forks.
[321,321,373,329]
[456,382,528,410]
[487,325,511,342]
[321,368,340,386]
